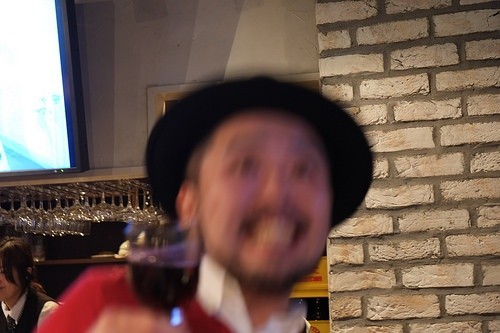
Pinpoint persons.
[0,236,60,333]
[34,73,374,333]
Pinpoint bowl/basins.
[118,240,131,256]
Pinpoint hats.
[146,76,372,227]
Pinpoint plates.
[91,255,114,258]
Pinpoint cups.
[31,238,45,262]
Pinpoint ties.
[6,314,16,333]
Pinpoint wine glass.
[0,180,161,237]
